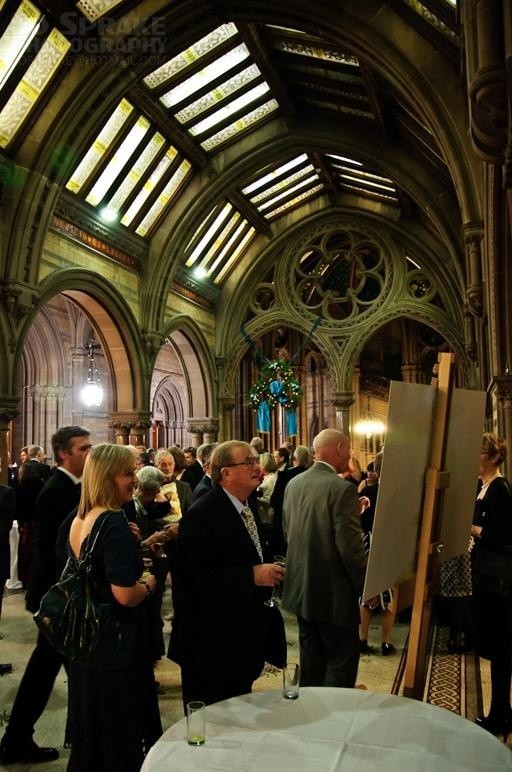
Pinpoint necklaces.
[481,473,500,488]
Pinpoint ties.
[242,506,264,564]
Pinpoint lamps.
[81,338,103,408]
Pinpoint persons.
[470,432,512,742]
[1,427,398,772]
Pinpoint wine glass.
[264,554,287,607]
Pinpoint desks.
[141,686,512,772]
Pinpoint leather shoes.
[0,734,59,766]
[360,639,368,652]
[382,642,395,655]
[0,664,12,674]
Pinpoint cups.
[282,662,299,699]
[187,700,207,744]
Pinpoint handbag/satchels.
[33,510,122,670]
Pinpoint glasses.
[219,456,260,473]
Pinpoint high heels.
[474,716,510,742]
